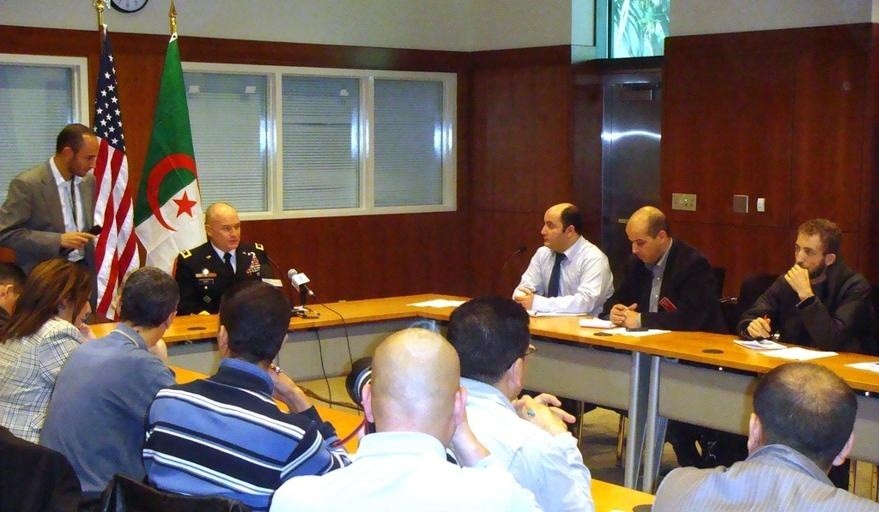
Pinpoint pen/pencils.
[526,408,535,416]
[764,314,767,321]
[627,327,648,332]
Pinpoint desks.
[0,294,879,512]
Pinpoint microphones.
[60,225,102,256]
[262,258,304,317]
[288,269,315,298]
[485,245,527,296]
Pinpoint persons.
[0,262,27,326]
[142,277,351,511]
[0,124,100,312]
[599,206,724,467]
[268,327,544,512]
[652,361,878,511]
[39,266,180,511]
[446,293,595,512]
[738,217,878,358]
[174,202,274,315]
[513,202,615,435]
[0,258,94,446]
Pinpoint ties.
[547,254,563,297]
[224,252,235,274]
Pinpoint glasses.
[524,343,537,357]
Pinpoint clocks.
[111,0,148,12]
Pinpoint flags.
[91,24,140,320]
[135,9,201,277]
